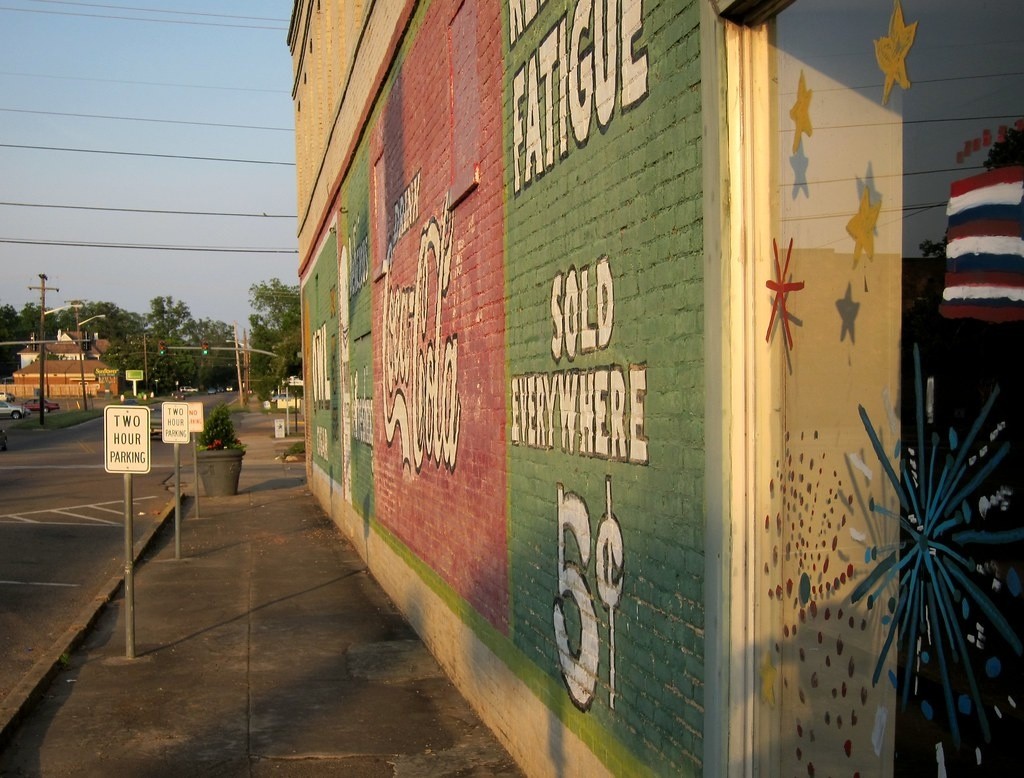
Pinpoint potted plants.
[196,399,245,498]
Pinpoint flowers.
[198,439,244,452]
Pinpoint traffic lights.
[203,342,209,355]
[159,340,166,355]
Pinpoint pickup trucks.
[179,386,199,393]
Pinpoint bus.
[1,377,14,385]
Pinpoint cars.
[0,392,15,403]
[0,429,7,452]
[217,387,224,393]
[24,397,61,414]
[207,387,217,395]
[16,406,31,415]
[0,401,24,420]
[226,385,233,392]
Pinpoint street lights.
[77,313,106,411]
[38,304,85,425]
[175,381,179,394]
[154,379,159,396]
